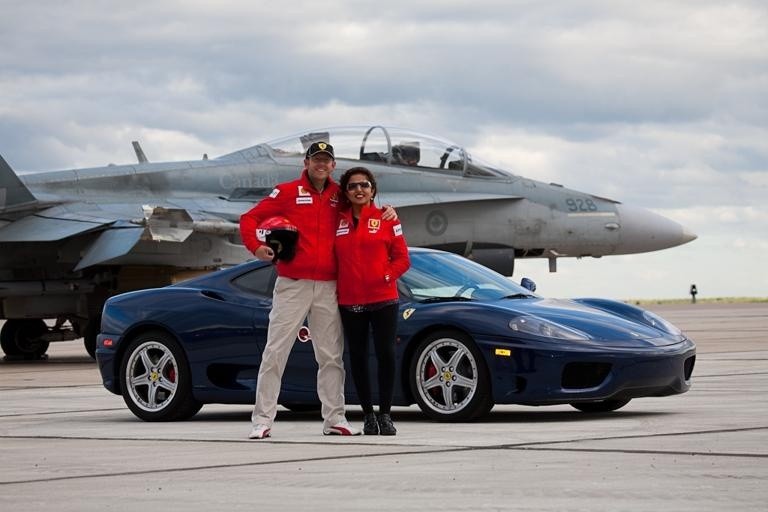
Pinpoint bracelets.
[384,271,392,285]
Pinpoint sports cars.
[94,245,699,423]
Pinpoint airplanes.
[1,125,700,366]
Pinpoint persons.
[334,166,411,436]
[239,141,400,439]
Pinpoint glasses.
[345,181,373,192]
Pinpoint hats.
[306,142,334,161]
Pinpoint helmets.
[256,215,298,265]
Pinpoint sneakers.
[364,412,379,435]
[378,411,396,435]
[249,424,271,438]
[323,420,362,436]
